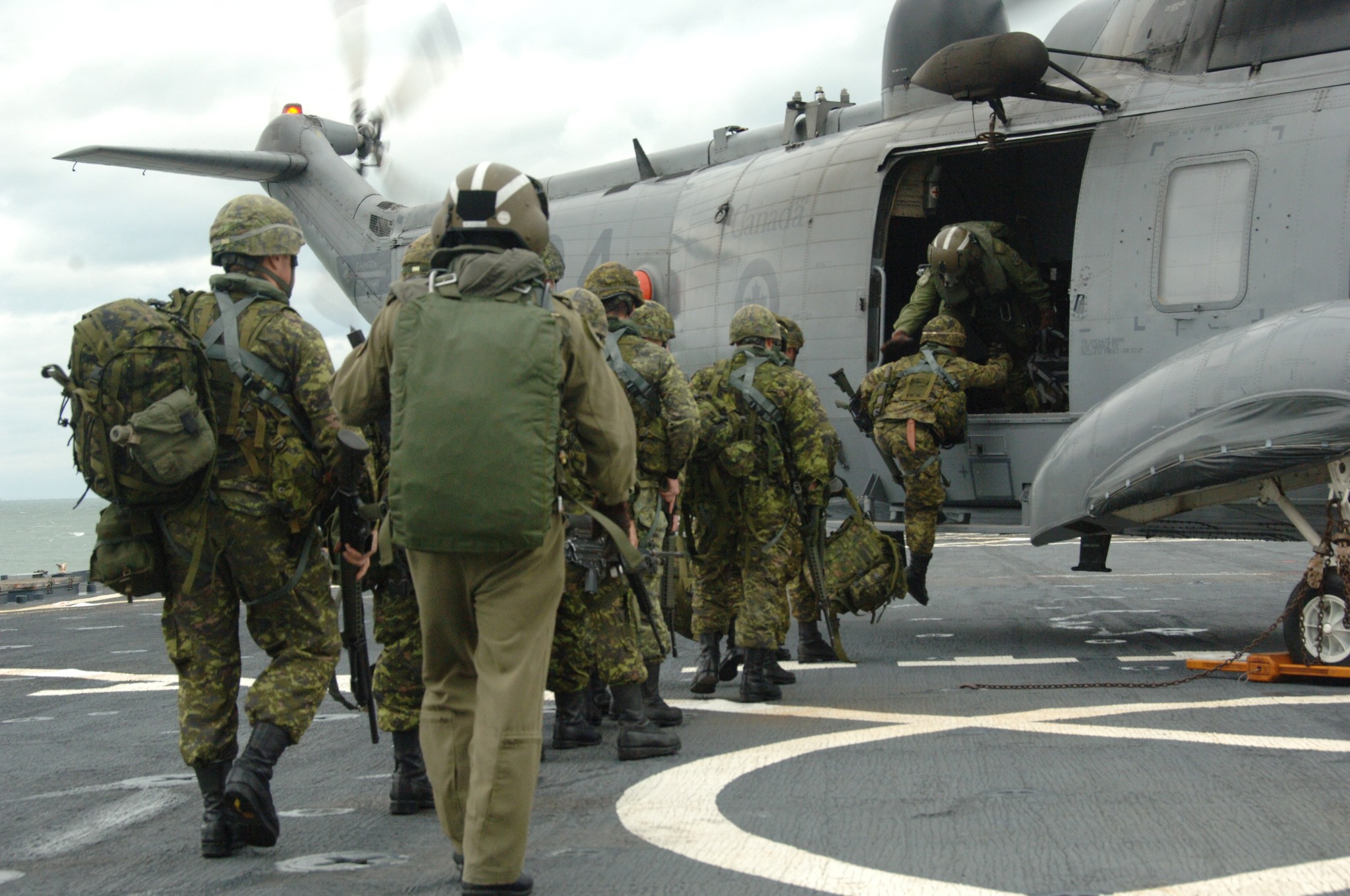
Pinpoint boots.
[188,544,937,856]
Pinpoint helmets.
[927,225,984,289]
[919,315,969,350]
[209,192,305,266]
[401,231,436,281]
[549,244,805,351]
[431,163,550,260]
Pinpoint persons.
[370,262,843,819]
[152,195,343,859]
[879,221,1054,415]
[329,163,639,895]
[859,313,1011,607]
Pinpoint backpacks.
[39,289,220,514]
[802,476,908,664]
[677,359,759,554]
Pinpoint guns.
[829,366,909,494]
[323,457,381,746]
[604,499,668,659]
[793,479,832,610]
[658,480,678,659]
[561,513,688,603]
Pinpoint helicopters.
[52,1,1350,676]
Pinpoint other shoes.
[461,869,534,895]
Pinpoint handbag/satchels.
[933,390,970,449]
[89,498,178,605]
